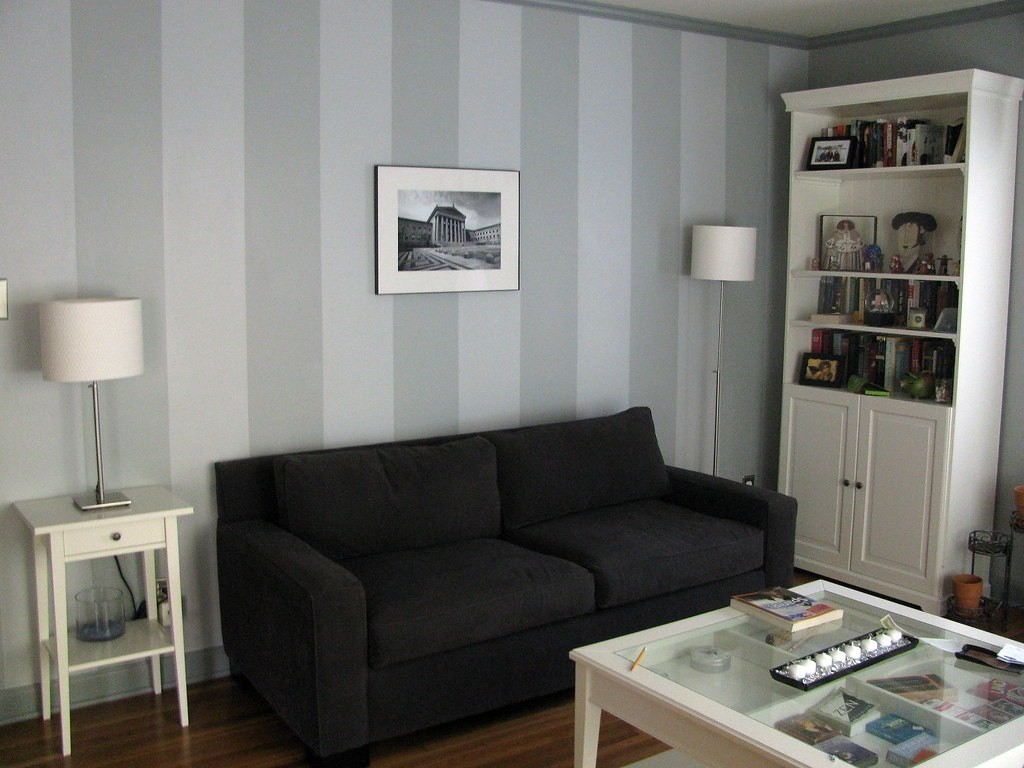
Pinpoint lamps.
[691,225,756,475]
[40,297,145,510]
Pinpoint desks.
[568,580,1024,768]
[13,486,194,756]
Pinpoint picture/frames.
[805,136,857,169]
[374,164,521,295]
[798,352,846,390]
[819,213,877,271]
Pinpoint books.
[774,667,1024,768]
[731,585,844,632]
[817,276,959,406]
[820,117,965,168]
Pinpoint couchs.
[214,406,797,768]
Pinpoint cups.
[75,587,125,641]
[952,574,984,616]
[1014,486,1024,526]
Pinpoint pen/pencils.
[787,629,818,653]
[629,646,648,671]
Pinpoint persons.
[816,145,841,162]
[812,360,833,380]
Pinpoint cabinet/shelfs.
[780,67,1024,615]
